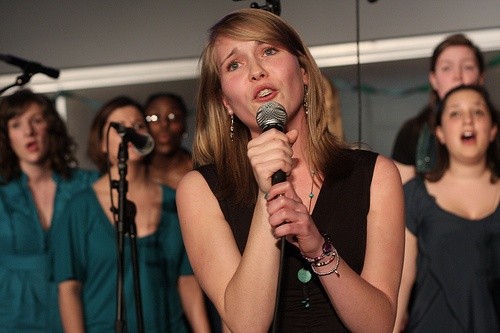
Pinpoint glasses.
[145,112,182,122]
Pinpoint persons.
[392,34,500,185]
[175,9,405,333]
[143,91,199,191]
[393,85,500,333]
[51,96,211,333]
[0,90,103,333]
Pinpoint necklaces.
[297,169,315,309]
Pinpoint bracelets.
[300,233,341,277]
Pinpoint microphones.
[256,102,289,237]
[109,121,155,157]
